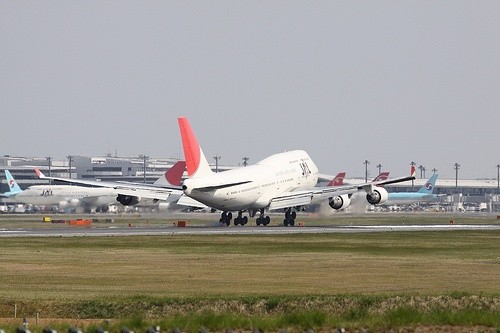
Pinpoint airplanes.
[2,117,454,225]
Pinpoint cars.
[371,205,425,211]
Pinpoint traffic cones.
[497,215,500,219]
[451,219,455,224]
